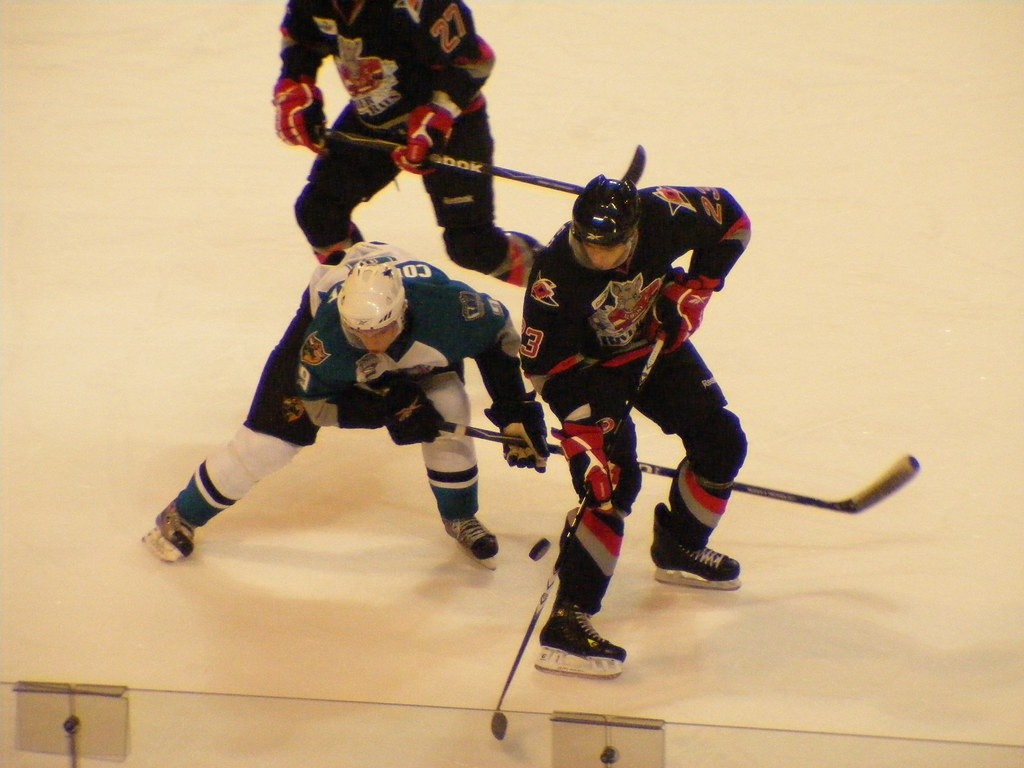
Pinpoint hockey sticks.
[488,327,672,743]
[320,126,650,200]
[435,418,925,519]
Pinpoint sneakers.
[650,503,742,590]
[442,516,499,571]
[533,595,626,675]
[142,497,195,560]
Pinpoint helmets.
[568,174,641,270]
[338,260,405,350]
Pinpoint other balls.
[528,535,551,562]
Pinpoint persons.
[140,239,551,569]
[272,0,549,288]
[519,173,752,678]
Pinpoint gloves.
[273,79,329,156]
[645,267,721,354]
[483,390,550,473]
[551,420,620,517]
[380,382,447,446]
[390,105,454,176]
